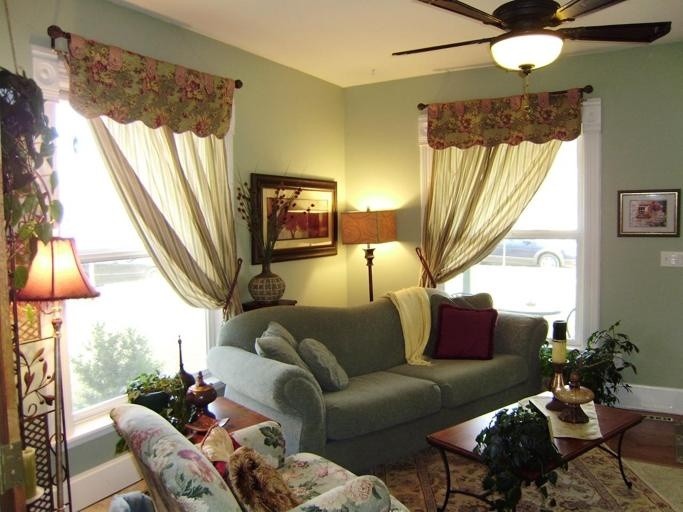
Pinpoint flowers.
[238,178,317,265]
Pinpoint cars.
[478,232,577,268]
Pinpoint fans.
[391,1,673,56]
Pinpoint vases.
[248,264,285,308]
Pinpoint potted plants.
[472,401,568,512]
[0,67,62,304]
[538,319,640,413]
[112,370,199,456]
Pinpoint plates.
[185,415,231,433]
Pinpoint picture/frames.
[617,188,681,238]
[248,172,339,266]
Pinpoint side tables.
[173,392,283,440]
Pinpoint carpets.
[357,441,678,511]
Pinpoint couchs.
[206,288,548,477]
[111,402,411,512]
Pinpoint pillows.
[433,304,496,360]
[201,425,244,480]
[229,445,301,512]
[253,337,323,397]
[429,292,493,357]
[298,338,349,392]
[261,322,299,353]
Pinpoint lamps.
[490,21,565,105]
[20,240,102,510]
[340,208,398,303]
